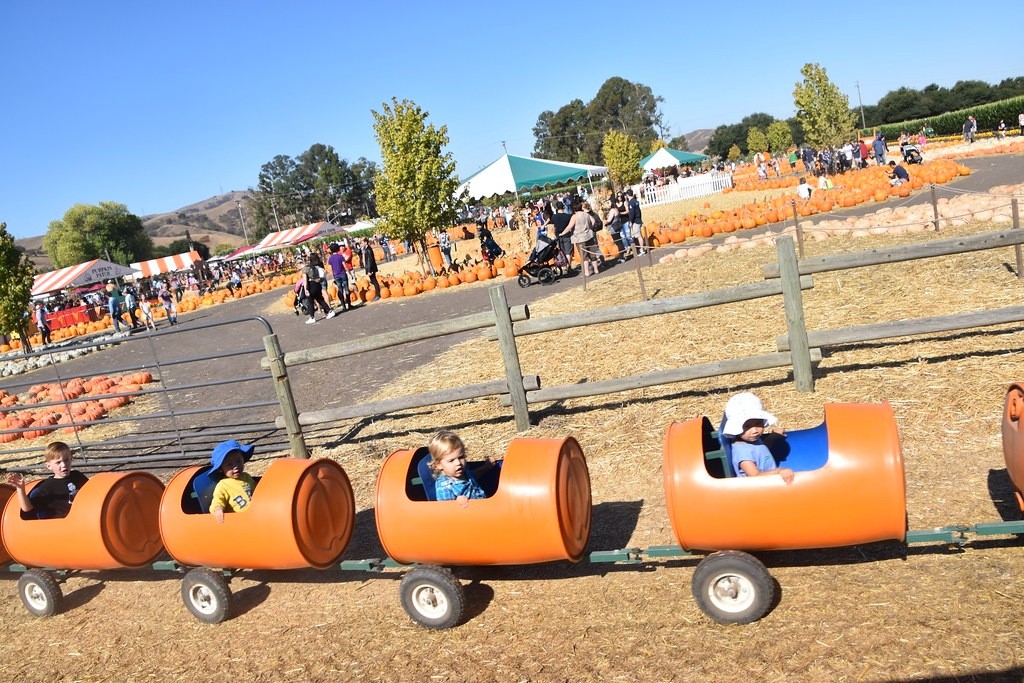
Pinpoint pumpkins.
[231,217,619,313]
[0,288,231,443]
[638,136,1024,262]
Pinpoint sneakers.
[305,317,316,324]
[326,311,335,319]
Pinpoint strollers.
[902,143,922,165]
[515,228,567,288]
[292,280,310,316]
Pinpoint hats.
[722,392,777,438]
[207,439,255,477]
[338,241,345,246]
[556,201,565,207]
[626,189,633,195]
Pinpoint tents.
[446,153,609,252]
[205,221,353,269]
[639,144,709,182]
[29,251,203,304]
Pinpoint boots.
[584,261,591,276]
[592,261,600,274]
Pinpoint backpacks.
[591,213,603,232]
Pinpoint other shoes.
[372,295,379,301]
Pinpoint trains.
[0,371,1024,624]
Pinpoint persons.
[541,187,646,279]
[426,431,496,508]
[23,275,184,347]
[754,123,936,180]
[722,392,794,485]
[885,161,910,187]
[177,251,306,298]
[207,439,255,524]
[640,168,670,203]
[378,197,544,266]
[294,233,380,324]
[6,442,89,519]
[667,159,751,184]
[796,170,842,199]
[962,111,1024,146]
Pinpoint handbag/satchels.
[440,246,445,252]
[315,265,327,279]
[554,249,567,267]
[612,221,623,231]
[631,227,635,238]
